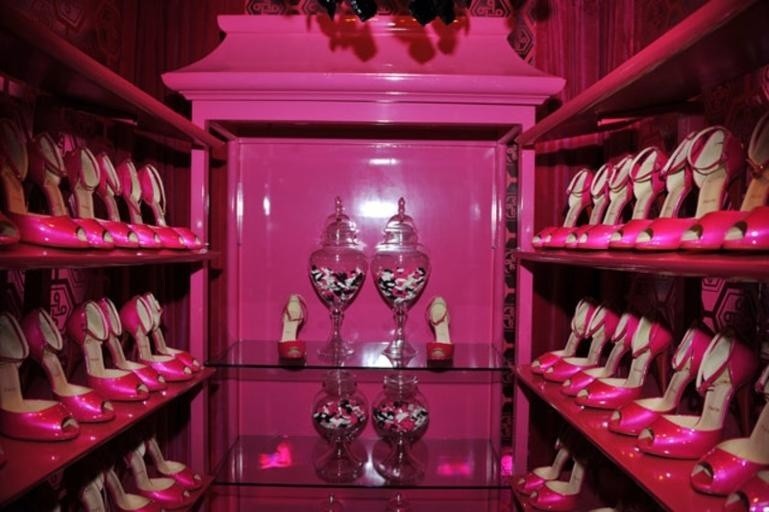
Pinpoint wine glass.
[307,196,429,480]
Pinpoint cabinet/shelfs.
[209,343,513,491]
[0,0,209,509]
[519,3,769,509]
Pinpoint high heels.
[1,131,209,248]
[514,434,590,496]
[65,416,203,512]
[277,294,308,359]
[529,301,768,511]
[531,113,769,250]
[0,292,206,442]
[424,296,454,360]
[529,450,627,512]
